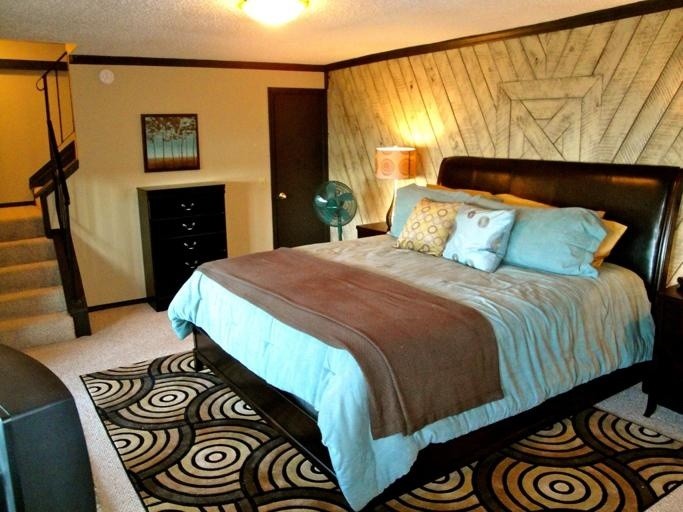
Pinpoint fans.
[315,180,358,241]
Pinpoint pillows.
[502,196,628,269]
[425,183,492,197]
[389,184,475,241]
[443,201,517,274]
[393,195,459,259]
[495,194,606,219]
[465,193,605,278]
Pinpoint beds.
[168,154,683,512]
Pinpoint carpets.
[78,349,683,512]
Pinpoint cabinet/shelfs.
[136,181,228,311]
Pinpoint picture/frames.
[140,111,200,175]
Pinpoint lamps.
[375,146,417,231]
[239,0,309,32]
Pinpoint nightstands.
[356,222,390,239]
[643,275,683,424]
